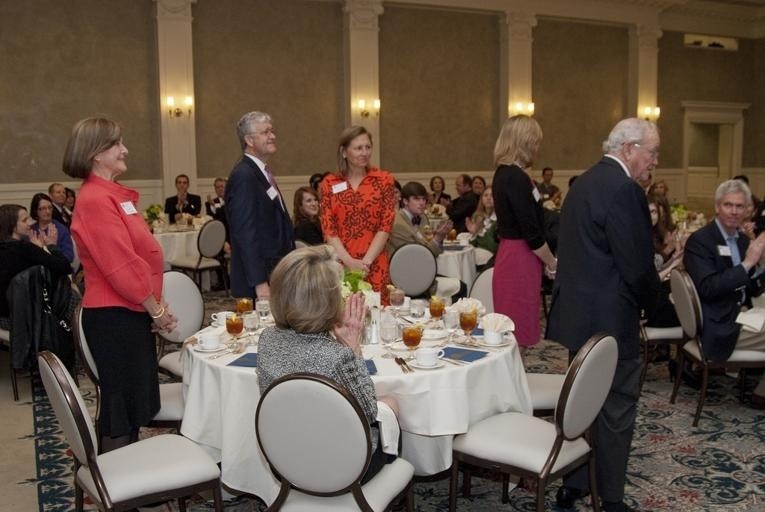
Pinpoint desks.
[180,293,535,510]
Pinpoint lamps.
[355,96,383,120]
[164,93,197,121]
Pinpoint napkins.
[477,313,515,333]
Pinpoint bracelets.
[149,306,164,319]
[544,257,557,274]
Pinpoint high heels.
[669,359,702,389]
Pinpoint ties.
[265,165,276,191]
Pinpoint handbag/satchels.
[55,288,80,332]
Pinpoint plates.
[406,357,447,369]
[443,238,471,253]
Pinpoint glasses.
[635,143,659,158]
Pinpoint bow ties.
[412,216,420,225]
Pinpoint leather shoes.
[556,485,590,508]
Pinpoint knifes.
[399,357,415,377]
[395,357,409,375]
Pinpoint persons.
[541,118,668,512]
[164,174,202,225]
[488,113,559,366]
[205,177,232,292]
[61,117,179,452]
[0,184,84,334]
[292,171,333,249]
[254,244,401,489]
[385,172,500,303]
[537,168,579,257]
[642,174,764,413]
[318,123,395,306]
[223,111,296,302]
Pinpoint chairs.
[500,374,566,498]
[150,268,206,382]
[37,349,224,511]
[665,264,764,428]
[1,204,764,314]
[72,298,186,451]
[635,302,700,402]
[447,332,621,508]
[255,373,417,511]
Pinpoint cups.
[677,213,710,232]
[150,210,213,236]
[411,348,445,365]
[193,289,515,360]
